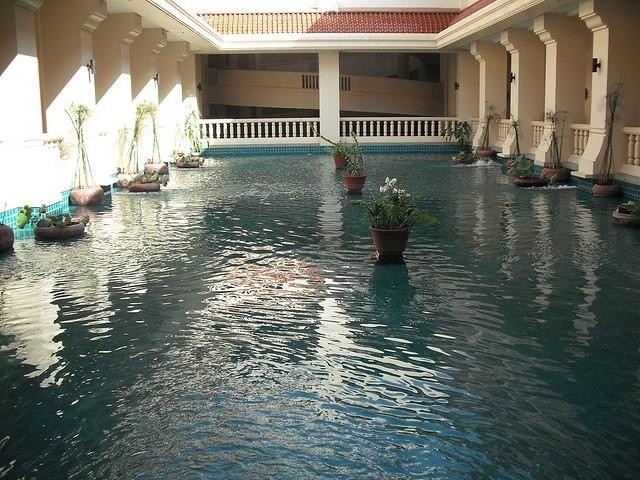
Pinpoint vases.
[369,224,411,254]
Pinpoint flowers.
[352,174,441,231]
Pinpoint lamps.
[87,58,96,76]
[591,58,602,73]
[152,73,161,86]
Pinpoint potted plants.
[310,122,368,191]
[442,73,626,194]
[16,99,205,240]
